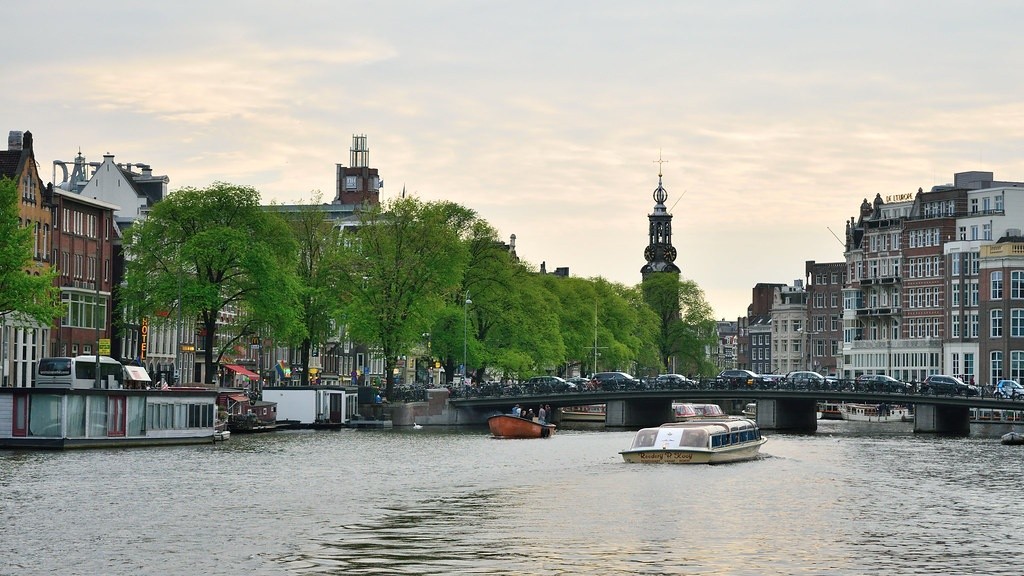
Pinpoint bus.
[34,355,124,389]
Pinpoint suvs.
[716,370,777,390]
[786,371,838,390]
[923,374,980,397]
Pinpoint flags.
[276,360,285,380]
[135,357,142,366]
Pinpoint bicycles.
[393,382,529,403]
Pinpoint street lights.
[463,290,473,379]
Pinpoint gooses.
[413,422,423,430]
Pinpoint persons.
[161,382,169,390]
[512,404,535,420]
[959,374,964,382]
[970,375,975,385]
[538,404,551,423]
[876,402,887,416]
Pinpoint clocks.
[644,246,655,261]
[663,245,677,262]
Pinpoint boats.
[816,402,909,422]
[561,405,605,422]
[618,415,768,464]
[672,402,757,422]
[487,413,556,439]
[1001,431,1024,444]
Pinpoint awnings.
[124,366,152,381]
[223,364,260,380]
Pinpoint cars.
[993,380,1024,400]
[656,374,700,390]
[528,372,646,393]
[857,374,912,393]
